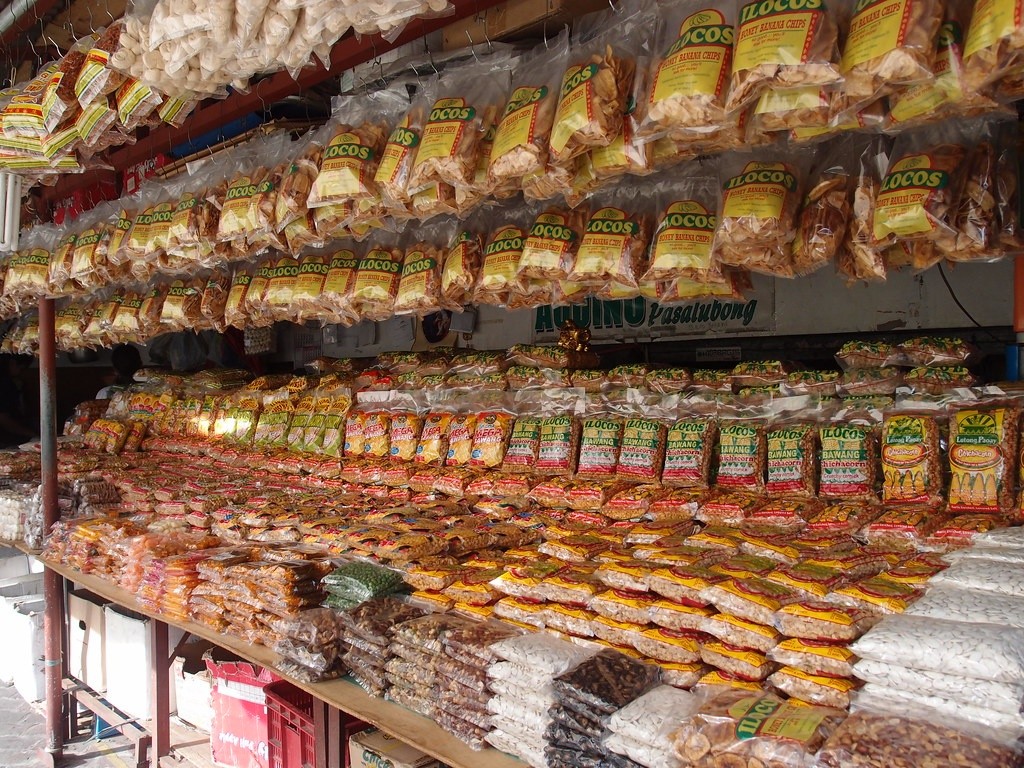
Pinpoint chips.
[0,0,1024,356]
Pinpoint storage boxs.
[208,649,283,768]
[262,679,363,768]
[106,602,202,721]
[0,544,44,704]
[173,639,214,732]
[71,588,112,698]
[348,726,445,768]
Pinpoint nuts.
[818,525,1024,768]
[269,600,706,768]
